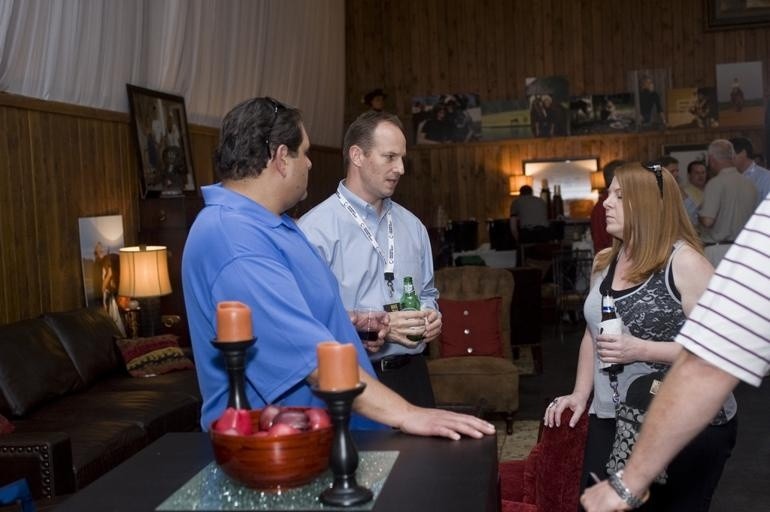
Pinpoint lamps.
[119,246,173,336]
[509,175,533,195]
[590,171,606,191]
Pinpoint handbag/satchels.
[607,403,669,486]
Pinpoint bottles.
[553,185,564,219]
[541,180,553,219]
[601,289,624,375]
[399,276,427,341]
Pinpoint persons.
[577,189,770,510]
[590,136,770,270]
[508,185,553,259]
[295,107,443,407]
[91,238,119,310]
[357,86,479,147]
[527,73,748,138]
[541,158,741,510]
[179,95,499,454]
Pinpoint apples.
[215,405,331,437]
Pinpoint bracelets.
[601,462,649,510]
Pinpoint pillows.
[114,335,194,377]
[436,296,503,359]
[0,414,15,434]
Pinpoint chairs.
[507,262,542,374]
[425,265,518,434]
[499,403,591,512]
[556,250,594,341]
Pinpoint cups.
[352,307,382,342]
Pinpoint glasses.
[264,95,284,160]
[643,157,665,197]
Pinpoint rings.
[549,400,555,408]
[554,402,560,407]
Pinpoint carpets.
[487,417,542,461]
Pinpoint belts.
[701,239,736,248]
[369,352,421,372]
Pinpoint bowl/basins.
[211,407,333,491]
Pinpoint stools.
[521,241,559,295]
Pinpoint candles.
[317,341,358,390]
[216,301,253,341]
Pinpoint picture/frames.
[704,0,770,33]
[126,81,197,198]
[662,144,708,183]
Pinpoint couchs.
[0,308,202,499]
